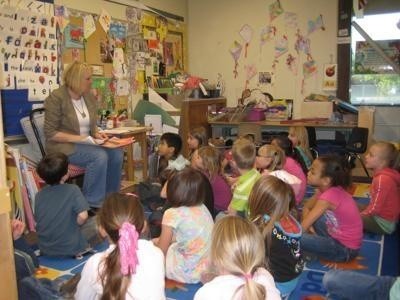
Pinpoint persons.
[34,127,399,300]
[43,61,123,216]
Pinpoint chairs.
[17,106,88,190]
[323,127,370,177]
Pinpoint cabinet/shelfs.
[163,94,227,158]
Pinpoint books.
[85,135,136,149]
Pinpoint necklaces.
[70,100,86,118]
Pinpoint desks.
[206,118,358,145]
[101,124,153,183]
